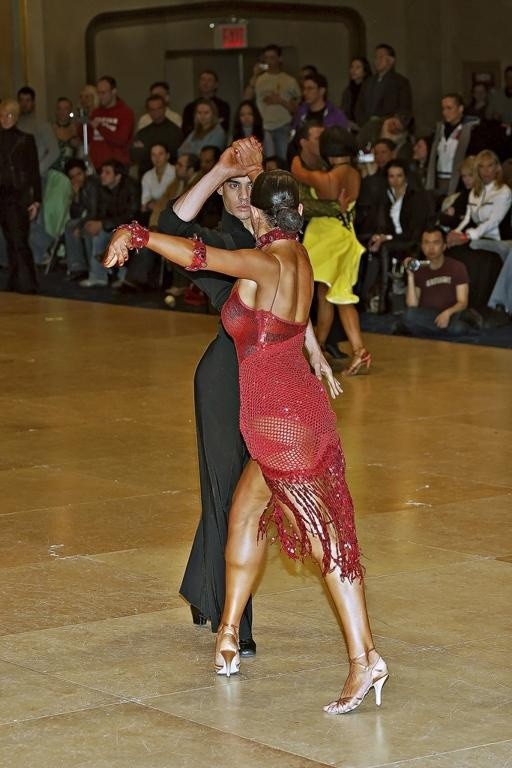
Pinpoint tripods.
[42,159,99,279]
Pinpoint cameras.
[358,153,375,163]
[259,63,268,69]
[410,258,430,269]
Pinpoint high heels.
[341,349,371,376]
[323,656,388,714]
[214,630,241,677]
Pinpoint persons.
[1,72,275,309]
[248,45,512,376]
[101,136,390,715]
[159,137,345,659]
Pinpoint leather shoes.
[211,619,256,657]
[324,343,346,358]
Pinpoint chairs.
[344,175,512,314]
